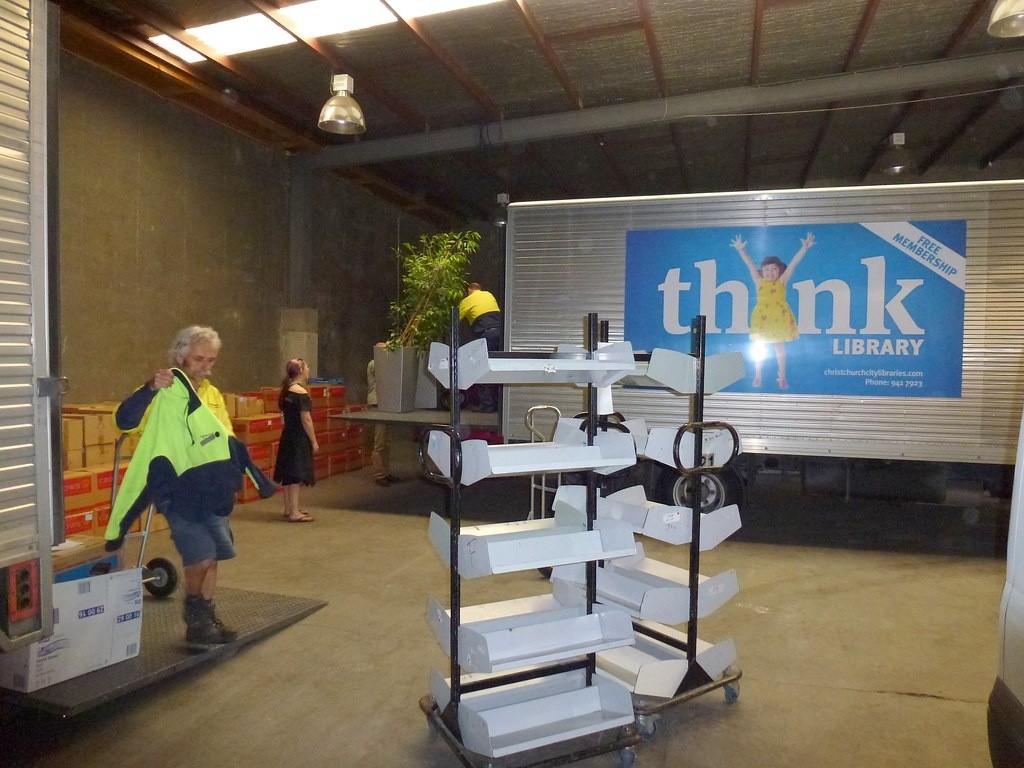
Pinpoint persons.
[367,343,402,487]
[458,283,502,413]
[272,358,319,522]
[727,232,819,389]
[113,324,249,647]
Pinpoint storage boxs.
[0,556,145,694]
[54,373,374,552]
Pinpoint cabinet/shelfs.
[548,315,746,740]
[422,305,649,768]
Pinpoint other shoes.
[378,477,391,485]
[383,472,400,484]
[776,374,790,389]
[753,376,762,387]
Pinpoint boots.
[202,603,238,644]
[182,599,222,652]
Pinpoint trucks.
[492,178,1024,522]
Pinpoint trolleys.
[51,430,181,599]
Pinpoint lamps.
[317,71,367,137]
[987,0,1023,39]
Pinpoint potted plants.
[373,229,482,413]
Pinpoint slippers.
[289,513,316,524]
[283,507,312,518]
